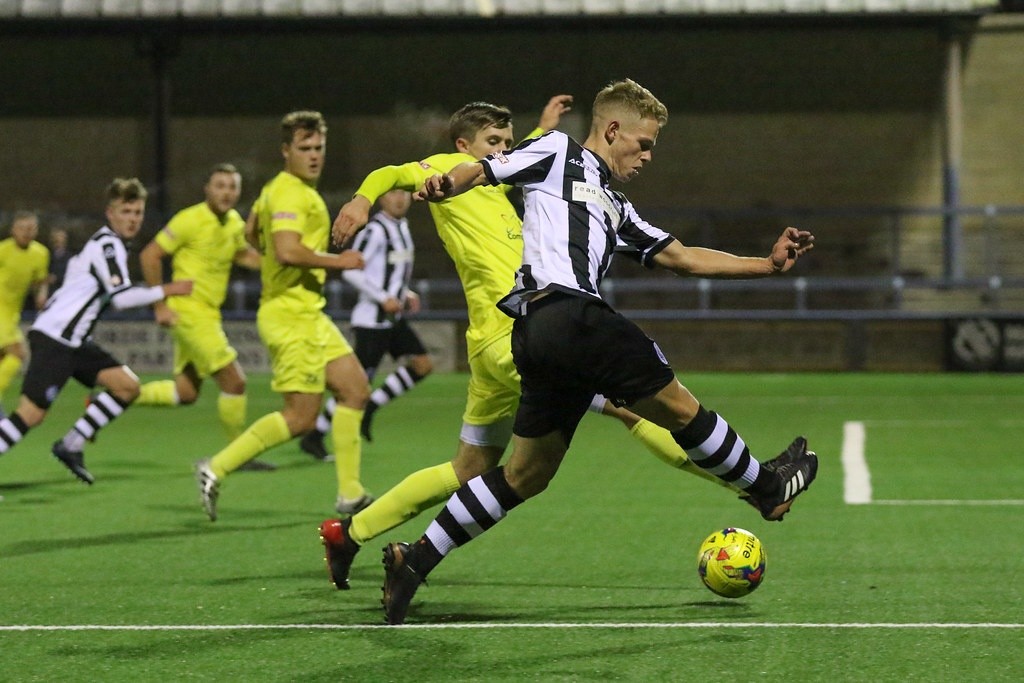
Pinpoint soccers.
[697,527,767,598]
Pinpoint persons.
[380,78,817,624]
[86,164,275,470]
[0,178,194,484]
[0,211,74,400]
[194,112,375,521]
[300,190,433,460]
[318,94,807,590]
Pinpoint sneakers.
[302,430,328,458]
[738,436,807,511]
[51,438,95,484]
[335,491,376,515]
[195,457,220,521]
[237,458,276,471]
[319,515,361,589]
[360,406,371,441]
[381,542,422,625]
[84,391,100,442]
[758,451,818,521]
